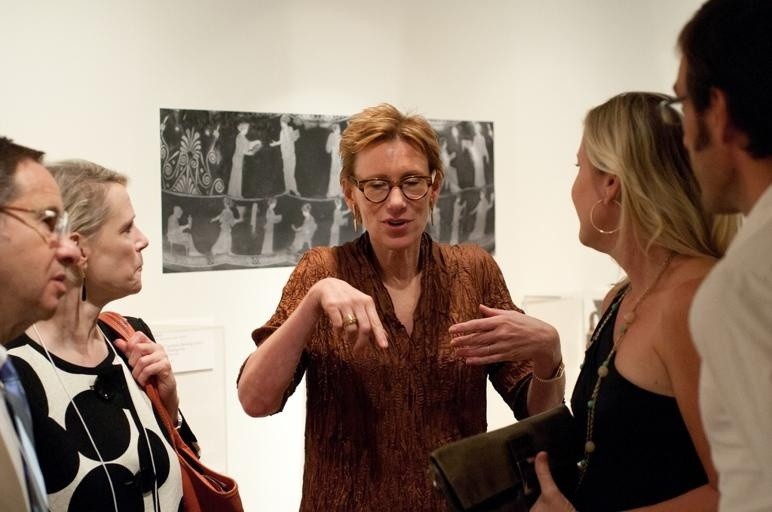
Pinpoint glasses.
[657,96,688,125]
[0,205,69,233]
[349,169,436,203]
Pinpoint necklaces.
[576,251,676,472]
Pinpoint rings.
[343,314,356,325]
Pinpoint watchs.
[535,358,565,383]
[175,411,183,429]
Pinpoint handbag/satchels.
[170,435,244,512]
[431,405,573,512]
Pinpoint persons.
[528,93,744,512]
[673,0,772,512]
[4,158,200,512]
[0,138,79,512]
[236,104,565,512]
